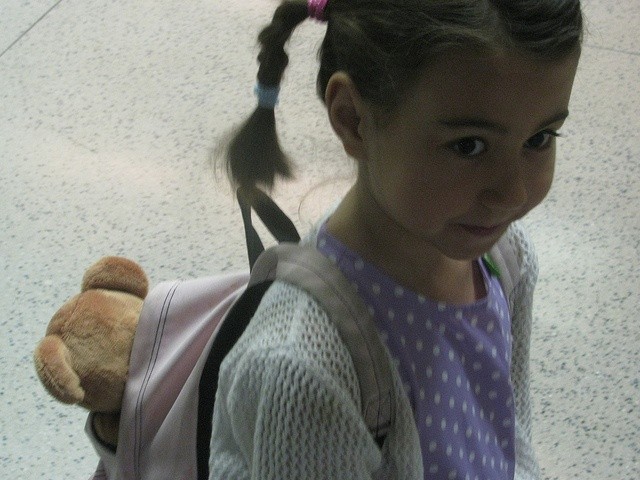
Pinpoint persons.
[206,0,585,480]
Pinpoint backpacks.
[85,183,522,480]
